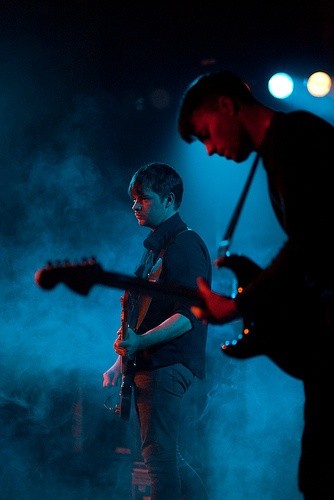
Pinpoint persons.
[101,164,213,499]
[177,76,334,499]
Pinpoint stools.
[131,469,156,500]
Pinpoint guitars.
[34,254,263,421]
